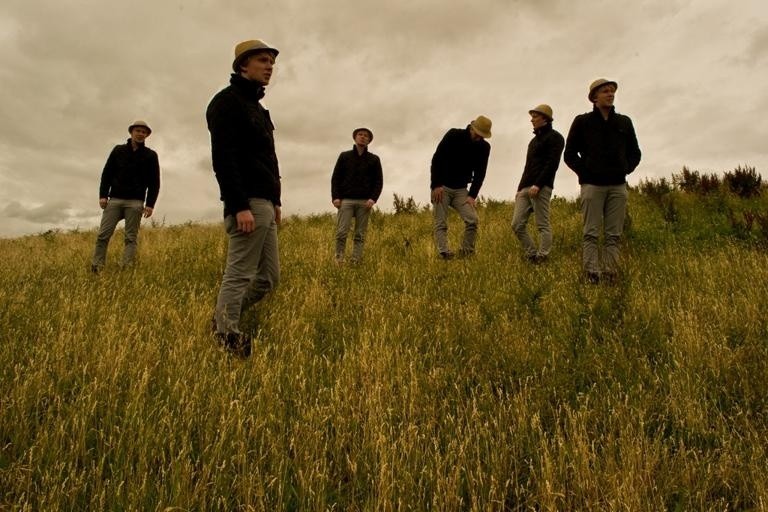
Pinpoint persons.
[89,119,159,275]
[430,115,492,259]
[510,105,564,266]
[207,38,281,360]
[331,128,383,268]
[563,79,642,284]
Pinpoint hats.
[233,40,279,72]
[528,104,553,121]
[588,79,617,102]
[128,121,151,137]
[470,115,492,138]
[352,128,373,144]
